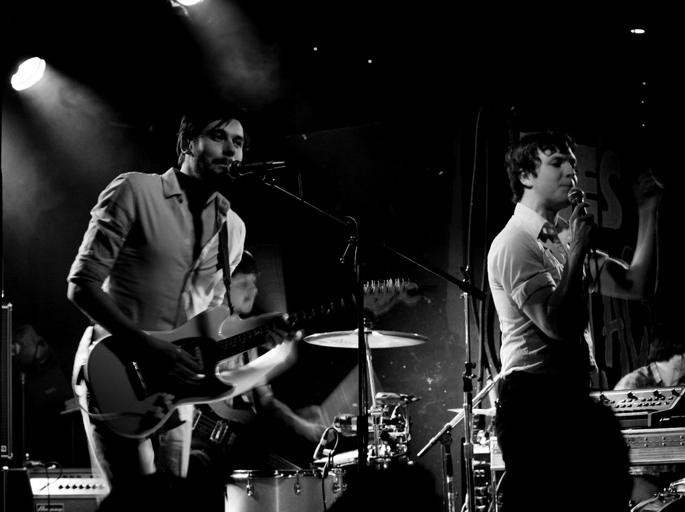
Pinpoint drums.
[222,469,345,512]
[312,445,416,508]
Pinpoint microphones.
[567,187,588,219]
[226,160,285,178]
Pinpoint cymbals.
[305,331,432,351]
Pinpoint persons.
[486,119,668,397]
[477,370,638,511]
[64,100,302,504]
[183,248,322,512]
[13,314,91,470]
[324,457,450,511]
[93,466,211,511]
[609,327,685,511]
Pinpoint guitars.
[84,280,420,440]
